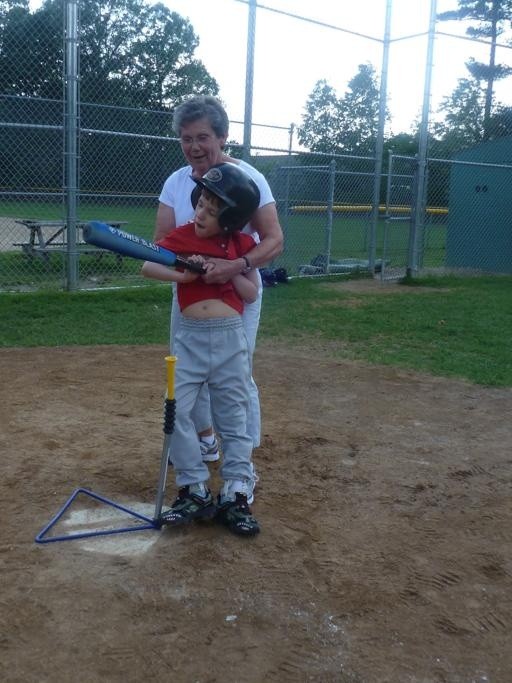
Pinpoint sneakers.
[158,440,261,535]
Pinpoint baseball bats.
[82,222,211,275]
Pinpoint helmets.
[189,162,260,233]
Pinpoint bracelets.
[241,254,253,274]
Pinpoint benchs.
[9,218,129,272]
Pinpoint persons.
[154,94,286,504]
[141,160,264,541]
